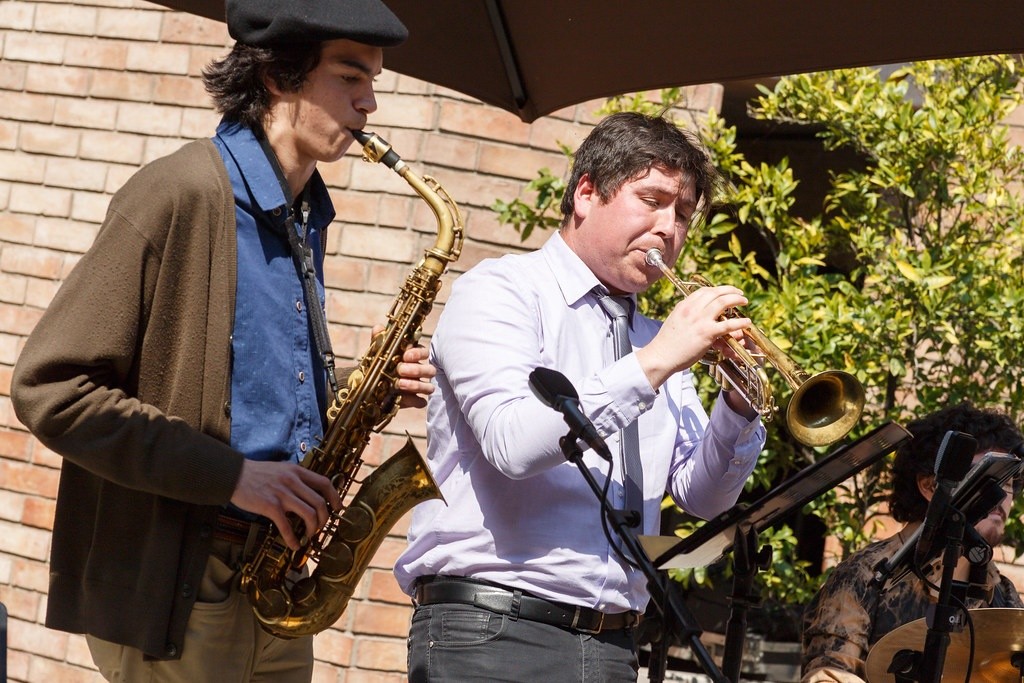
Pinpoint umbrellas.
[151,1,1024,126]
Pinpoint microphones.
[529,367,612,460]
[914,430,981,568]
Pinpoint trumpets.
[645,246,866,448]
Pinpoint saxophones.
[236,129,468,638]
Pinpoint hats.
[225,0,410,50]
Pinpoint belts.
[415,580,641,632]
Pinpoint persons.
[11,0,436,683]
[393,112,766,682]
[802,404,1024,683]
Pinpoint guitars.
[862,606,1024,683]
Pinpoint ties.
[595,289,644,566]
